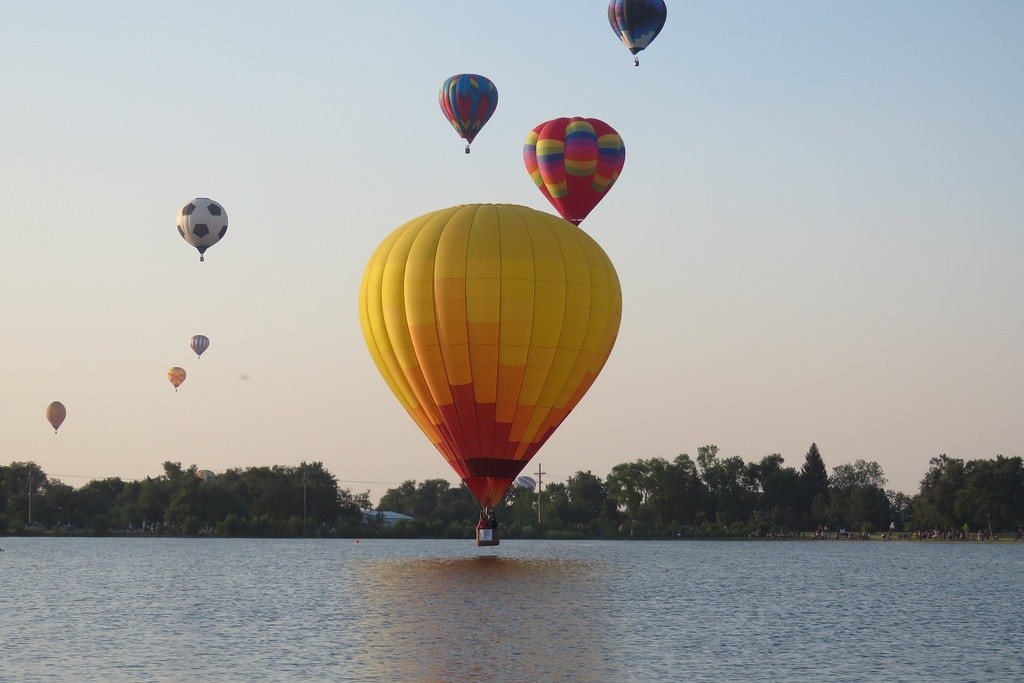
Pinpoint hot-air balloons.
[514,476,536,496]
[176,198,229,262]
[190,335,210,360]
[46,401,66,434]
[359,202,623,547]
[438,74,499,154]
[523,116,626,228]
[608,0,667,67]
[167,366,187,392]
